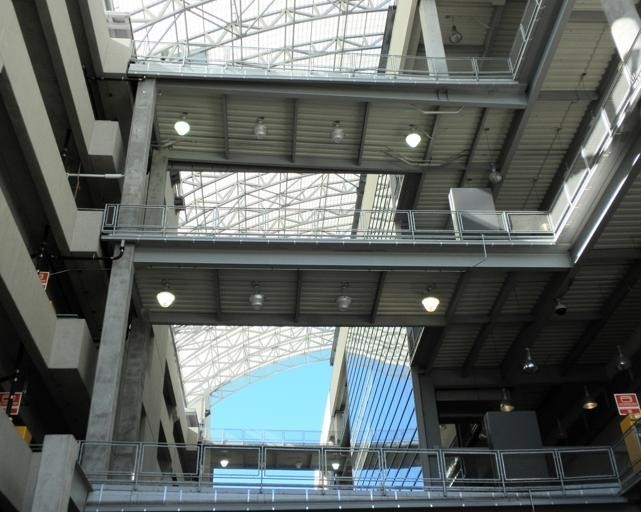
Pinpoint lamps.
[156,277,176,309]
[334,282,354,312]
[255,114,269,141]
[174,112,193,136]
[420,285,441,313]
[249,280,266,311]
[330,120,345,145]
[446,15,462,44]
[403,124,423,148]
[500,297,631,413]
[484,127,502,184]
